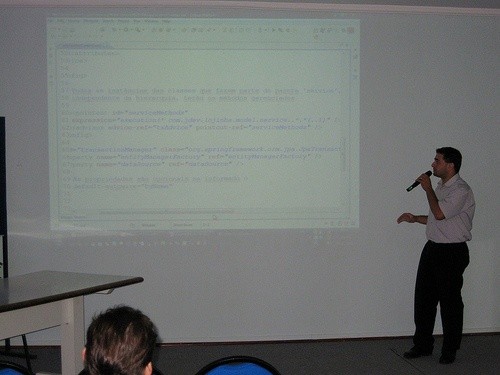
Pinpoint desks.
[0,270,144,375]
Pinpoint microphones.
[406,170,432,191]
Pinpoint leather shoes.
[403,345,433,358]
[439,355,454,364]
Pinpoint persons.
[397,147,476,365]
[82,305,161,375]
